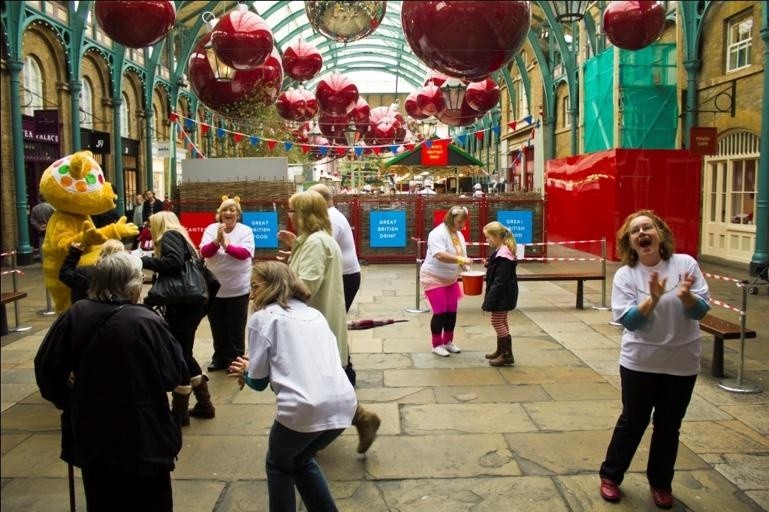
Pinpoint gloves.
[457,256,473,266]
[462,265,471,272]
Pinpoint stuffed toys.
[38,150,141,317]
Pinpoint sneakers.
[443,342,460,353]
[207,363,223,370]
[650,484,673,508]
[431,344,451,356]
[600,476,621,502]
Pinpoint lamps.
[204,3,235,81]
[305,1,384,40]
[440,75,465,119]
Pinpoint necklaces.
[451,232,460,245]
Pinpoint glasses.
[248,280,268,290]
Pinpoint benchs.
[695,312,757,380]
[454,270,606,310]
[0,290,33,333]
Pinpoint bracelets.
[456,256,464,265]
[219,238,228,246]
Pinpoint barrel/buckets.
[497,167,514,184]
[459,270,486,295]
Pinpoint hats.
[217,195,241,213]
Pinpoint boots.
[485,334,514,365]
[171,383,192,426]
[190,373,214,418]
[352,402,380,454]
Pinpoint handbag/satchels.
[143,230,209,306]
[59,396,84,466]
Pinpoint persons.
[275,182,361,313]
[228,260,357,511]
[59,238,127,304]
[286,190,381,454]
[198,196,256,373]
[34,253,191,511]
[598,208,710,509]
[29,189,166,251]
[419,205,474,357]
[480,221,518,366]
[342,182,486,198]
[141,209,215,425]
